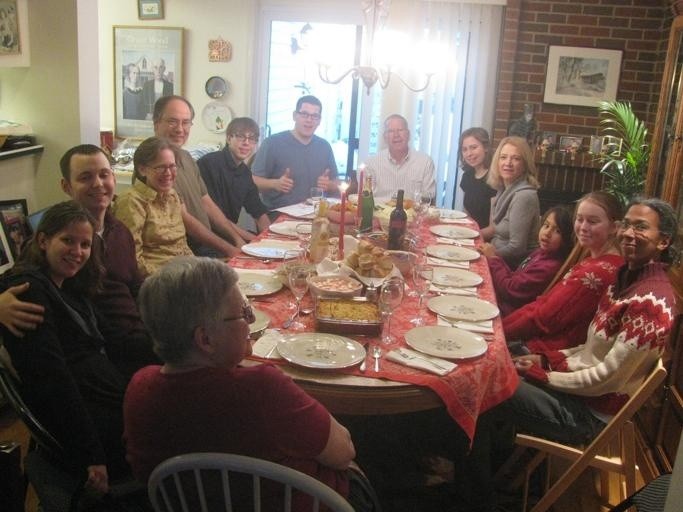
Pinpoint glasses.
[224,305,257,324]
[236,133,257,142]
[296,110,320,120]
[148,164,179,174]
[613,220,663,235]
[165,117,193,129]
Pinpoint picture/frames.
[109,23,186,144]
[0,0,31,69]
[541,129,624,159]
[134,0,167,20]
[0,198,31,277]
[539,42,624,112]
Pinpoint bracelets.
[540,355,548,371]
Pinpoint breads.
[345,240,393,278]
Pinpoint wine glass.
[309,185,323,218]
[381,279,405,346]
[284,222,312,331]
[412,193,432,325]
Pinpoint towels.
[385,344,455,378]
[428,216,476,297]
[251,327,289,362]
[435,311,496,336]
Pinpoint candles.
[353,163,369,226]
[335,179,352,263]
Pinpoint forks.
[392,347,448,372]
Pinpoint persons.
[458,132,497,231]
[447,193,680,510]
[479,136,544,268]
[106,134,198,276]
[130,97,246,262]
[196,115,271,242]
[474,202,576,322]
[144,58,175,115]
[120,254,388,512]
[0,200,140,495]
[123,63,145,121]
[364,115,437,208]
[249,96,344,209]
[0,143,167,372]
[498,191,625,370]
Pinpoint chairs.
[513,360,669,511]
[0,342,134,511]
[143,452,362,510]
[545,242,588,298]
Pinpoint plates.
[203,75,229,99]
[403,326,487,359]
[236,272,284,296]
[274,332,366,371]
[437,208,467,218]
[269,222,314,236]
[200,102,233,133]
[430,225,480,238]
[240,242,300,259]
[247,309,270,336]
[422,267,482,288]
[425,245,481,260]
[427,296,499,320]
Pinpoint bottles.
[360,175,374,229]
[387,188,406,250]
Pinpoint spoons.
[371,344,386,373]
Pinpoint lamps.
[310,1,443,95]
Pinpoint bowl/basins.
[315,295,384,335]
[309,276,364,297]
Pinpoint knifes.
[359,342,369,372]
